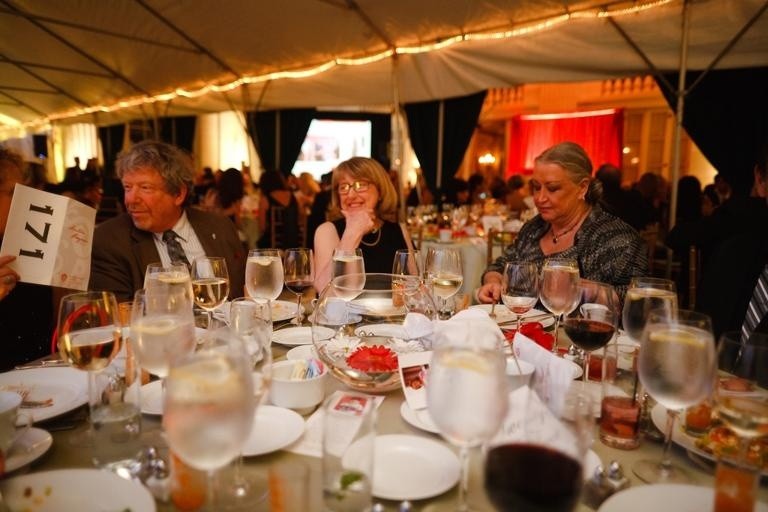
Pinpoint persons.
[193,166,332,251]
[65,157,104,205]
[598,156,767,383]
[388,169,536,217]
[477,141,654,336]
[314,157,420,300]
[87,139,249,331]
[1,143,74,375]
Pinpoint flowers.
[344,339,401,375]
[504,321,555,353]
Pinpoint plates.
[0,299,768,511]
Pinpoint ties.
[163,229,201,297]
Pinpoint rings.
[5,275,13,287]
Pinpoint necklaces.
[361,227,381,246]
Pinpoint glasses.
[338,181,373,196]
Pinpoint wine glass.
[55,246,768,512]
[406,198,538,246]
